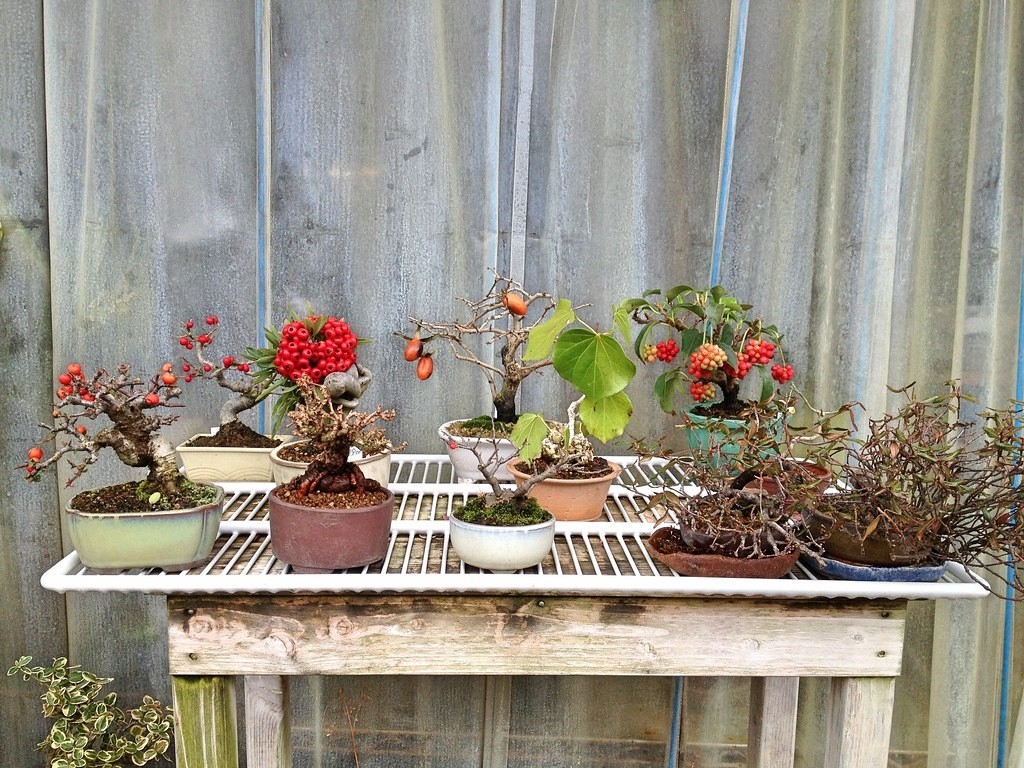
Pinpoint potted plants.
[12,362,225,573]
[738,381,832,495]
[506,295,636,520]
[242,301,393,489]
[176,317,293,482]
[394,267,592,480]
[821,375,1024,603]
[620,284,794,477]
[617,398,865,577]
[268,373,395,569]
[446,413,593,571]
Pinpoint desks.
[166,474,906,768]
